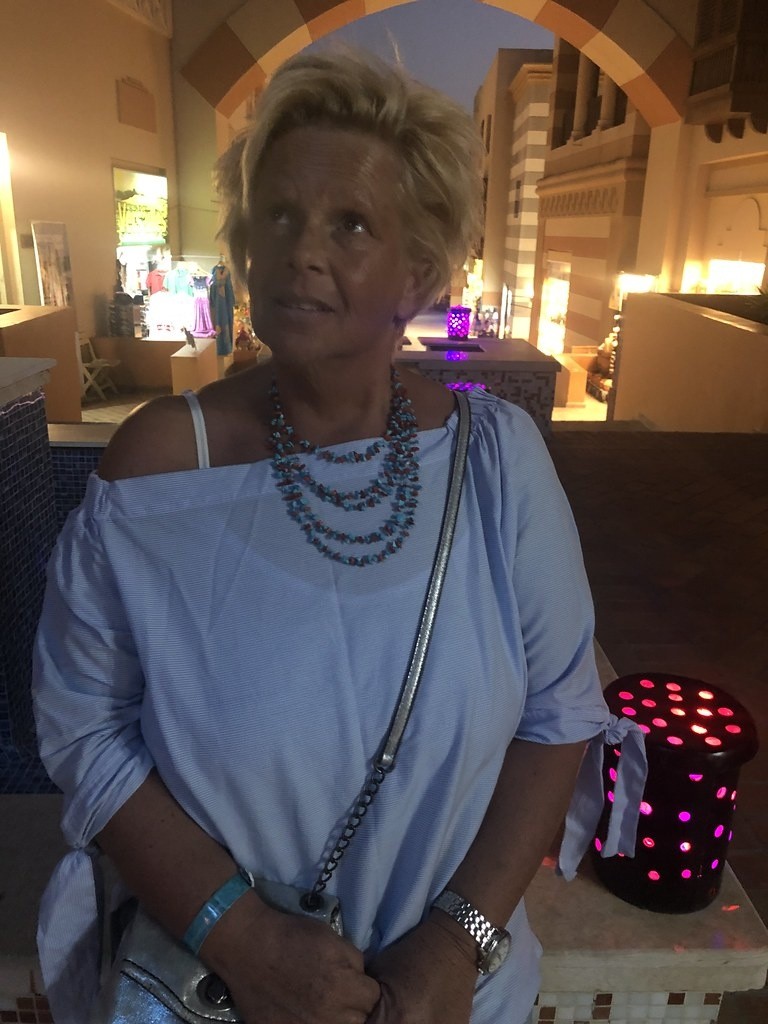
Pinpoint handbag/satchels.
[99,870,344,1024]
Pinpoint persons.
[29,44,649,1024]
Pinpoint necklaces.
[270,366,420,567]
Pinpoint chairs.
[75,330,122,401]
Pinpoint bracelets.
[183,867,254,957]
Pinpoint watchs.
[434,890,512,975]
